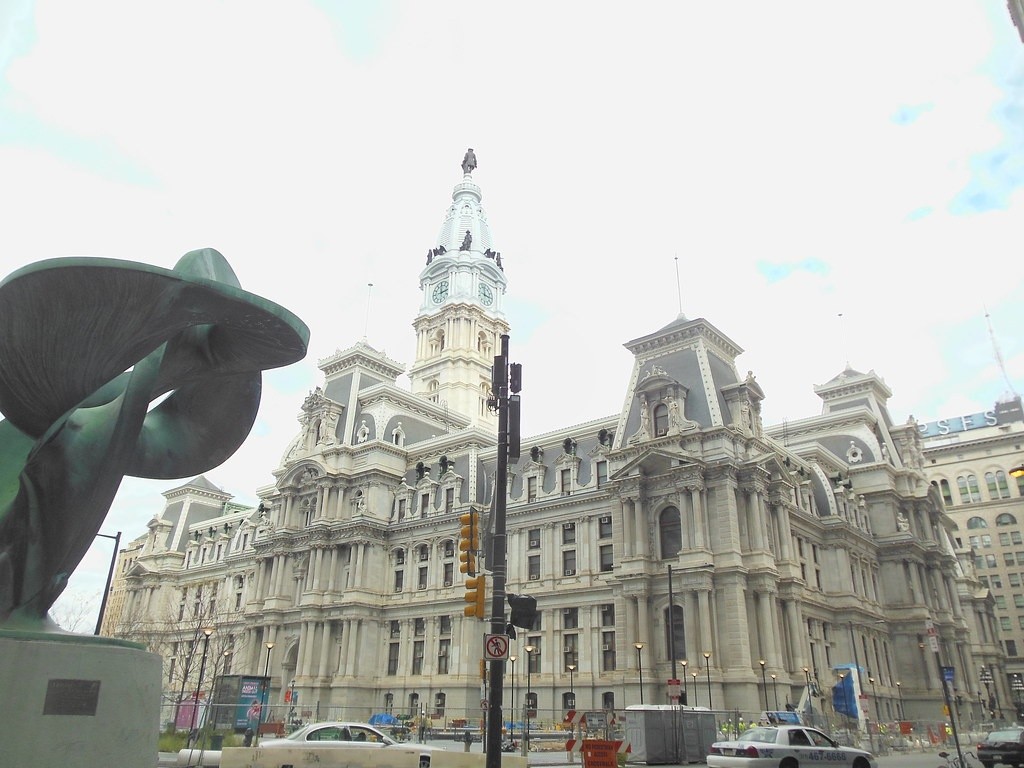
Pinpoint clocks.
[477,282,494,307]
[432,281,449,302]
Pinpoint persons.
[741,400,751,428]
[897,513,909,531]
[426,249,432,266]
[721,717,757,735]
[419,711,433,741]
[640,404,650,431]
[876,720,900,737]
[460,230,472,250]
[496,252,503,266]
[461,148,478,173]
[668,397,678,427]
[945,723,954,746]
[747,371,756,381]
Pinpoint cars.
[706,710,879,768]
[977,729,1024,768]
[259,722,446,751]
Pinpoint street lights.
[758,660,814,728]
[848,620,906,720]
[679,652,712,711]
[566,665,577,733]
[254,642,277,746]
[522,645,536,751]
[288,680,296,724]
[509,655,519,742]
[979,664,1024,721]
[186,629,216,750]
[212,651,230,731]
[632,642,647,704]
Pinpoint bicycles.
[938,752,978,768]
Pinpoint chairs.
[793,731,807,745]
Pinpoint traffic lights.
[460,506,478,556]
[464,576,485,620]
[460,551,476,578]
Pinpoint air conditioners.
[438,651,446,656]
[658,428,666,435]
[601,518,610,524]
[564,524,573,529]
[397,558,403,563]
[418,585,426,589]
[396,587,401,592]
[530,541,538,546]
[601,605,610,611]
[602,645,611,651]
[564,609,570,614]
[414,699,417,704]
[436,699,444,705]
[564,647,572,652]
[416,651,422,657]
[530,575,538,579]
[420,554,427,559]
[560,492,566,497]
[444,581,451,586]
[568,700,575,705]
[525,700,534,705]
[565,570,574,576]
[534,648,540,654]
[529,498,536,503]
[446,550,454,556]
